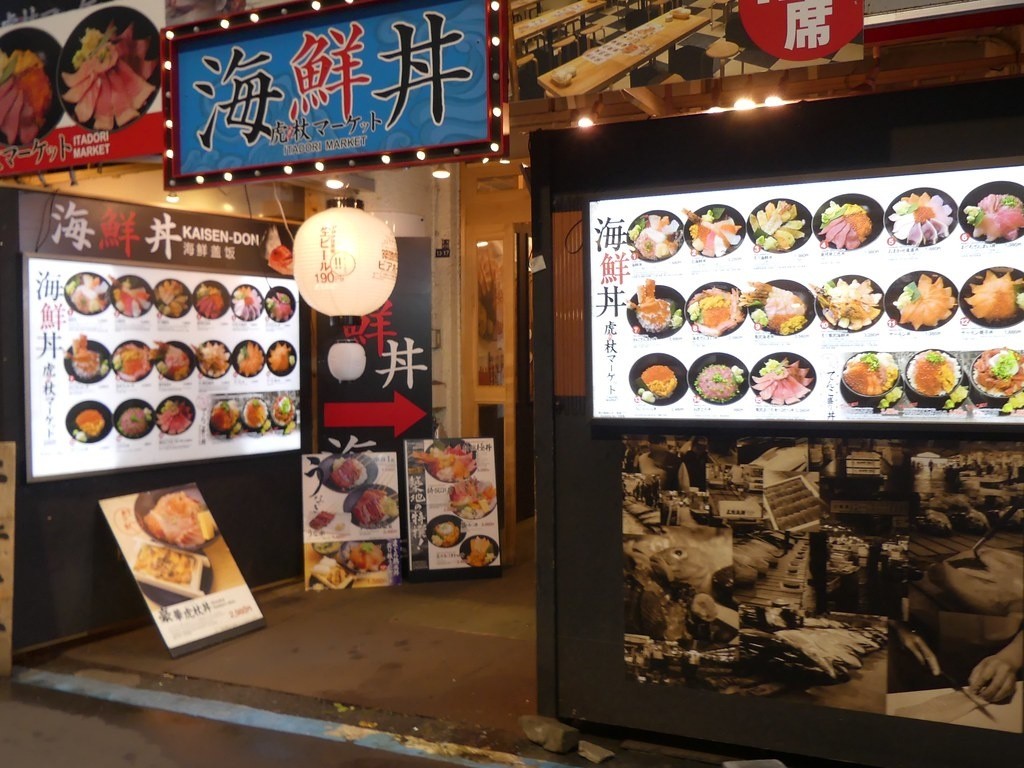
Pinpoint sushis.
[629,192,1024,413]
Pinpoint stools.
[512,0,674,78]
[703,39,746,79]
[647,72,682,86]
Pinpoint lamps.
[706,81,723,114]
[577,94,604,128]
[734,75,757,110]
[765,70,789,107]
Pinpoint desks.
[511,0,541,17]
[512,0,607,53]
[537,6,712,99]
